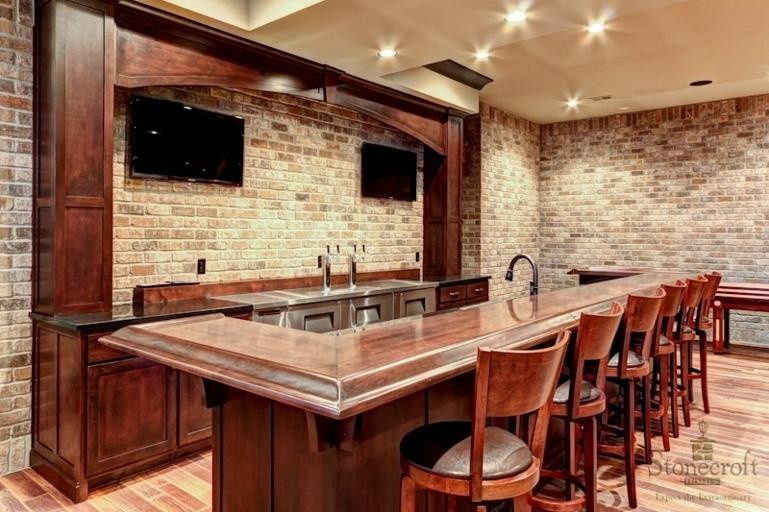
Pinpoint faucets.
[508,296,539,325]
[348,252,362,290]
[322,252,337,289]
[505,252,538,295]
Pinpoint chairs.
[398,272,722,511]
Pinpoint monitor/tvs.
[130,95,245,187]
[362,142,418,202]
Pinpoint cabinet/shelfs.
[24,322,214,506]
